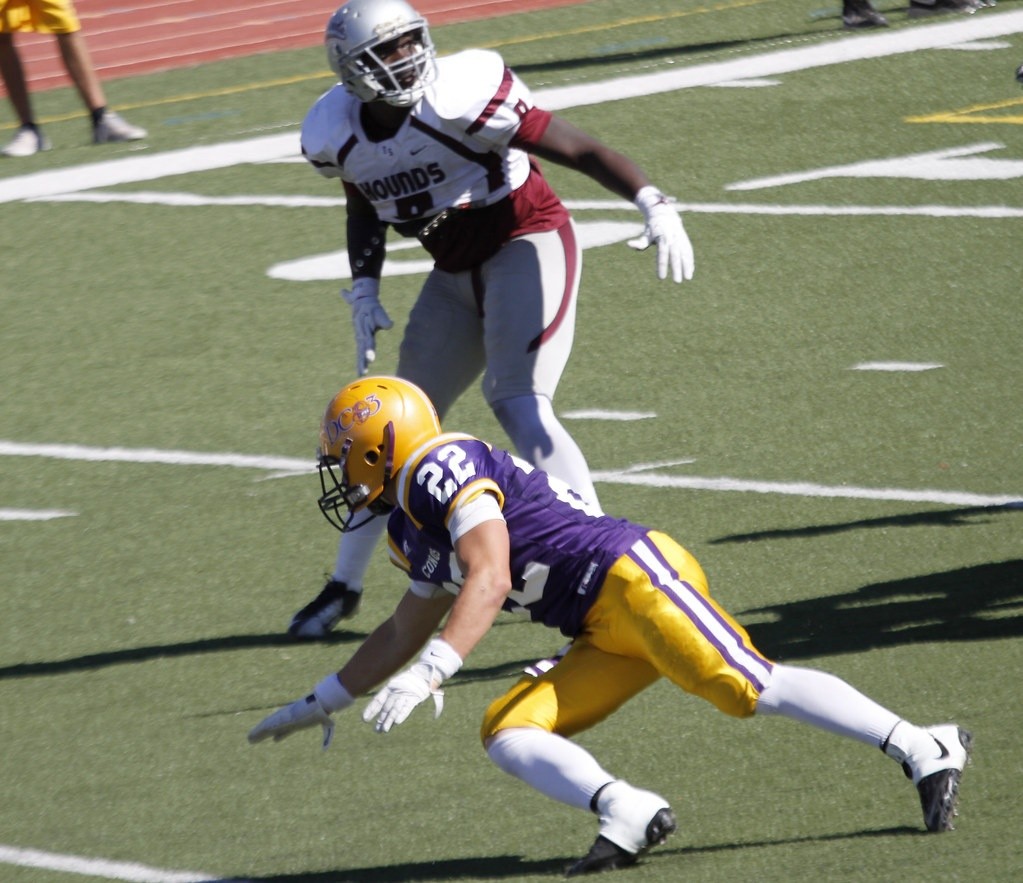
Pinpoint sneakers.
[521,640,574,678]
[565,780,676,877]
[289,573,362,638]
[902,724,973,832]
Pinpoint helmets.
[326,0,439,108]
[317,375,441,533]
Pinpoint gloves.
[342,278,394,375]
[248,675,355,750]
[361,638,464,736]
[626,185,694,283]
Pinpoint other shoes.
[92,106,148,144]
[908,0,975,18]
[842,4,889,31]
[0,122,54,156]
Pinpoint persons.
[246,372,973,877]
[281,0,698,639]
[0,0,148,159]
[841,0,997,28]
